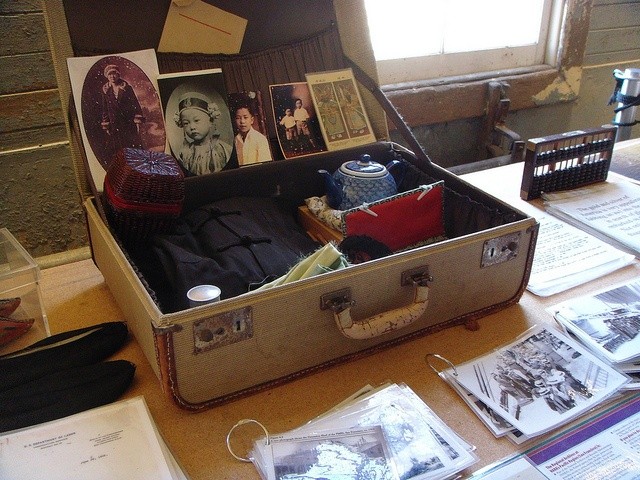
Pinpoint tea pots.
[317,153,405,211]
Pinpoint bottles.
[186,285,220,306]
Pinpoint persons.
[293,98,316,150]
[101,62,144,153]
[174,88,234,175]
[235,99,274,166]
[279,106,299,151]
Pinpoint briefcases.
[40,1,537,413]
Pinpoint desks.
[2,138,639,476]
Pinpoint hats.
[103,64,121,77]
[173,92,221,127]
